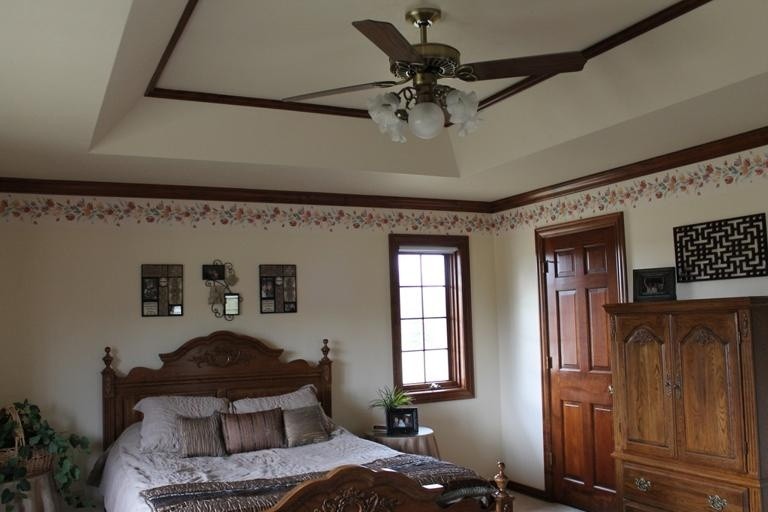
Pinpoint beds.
[95,329,514,511]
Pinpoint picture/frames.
[631,265,678,302]
[384,407,420,436]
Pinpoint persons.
[398,418,405,427]
[392,416,399,428]
[404,416,412,428]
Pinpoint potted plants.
[0,398,98,511]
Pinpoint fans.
[280,8,593,105]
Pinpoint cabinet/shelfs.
[601,294,768,511]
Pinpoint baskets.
[0,404,53,480]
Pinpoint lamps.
[365,71,483,146]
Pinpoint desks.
[368,425,435,455]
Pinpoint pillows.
[133,383,337,459]
